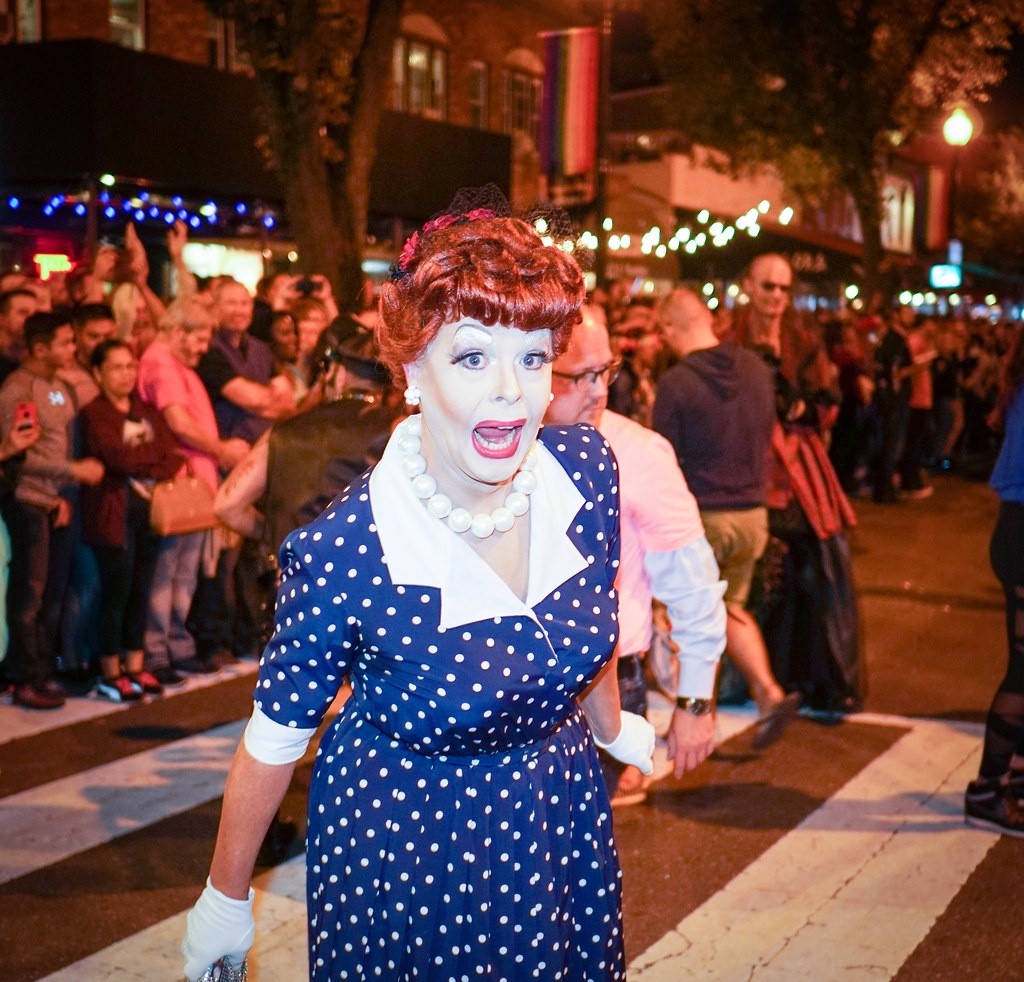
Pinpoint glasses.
[760,280,791,294]
[552,358,623,391]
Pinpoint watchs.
[677,697,713,715]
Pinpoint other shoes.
[95,670,164,704]
[172,661,220,675]
[608,770,648,805]
[209,650,239,667]
[151,667,186,689]
[12,682,68,709]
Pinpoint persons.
[0,217,1023,837]
[544,302,727,800]
[183,206,658,982]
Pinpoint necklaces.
[400,417,537,540]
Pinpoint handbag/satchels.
[150,451,223,536]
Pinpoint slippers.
[749,692,798,749]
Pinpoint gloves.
[180,878,256,981]
[592,709,655,777]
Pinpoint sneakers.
[965,772,1024,836]
